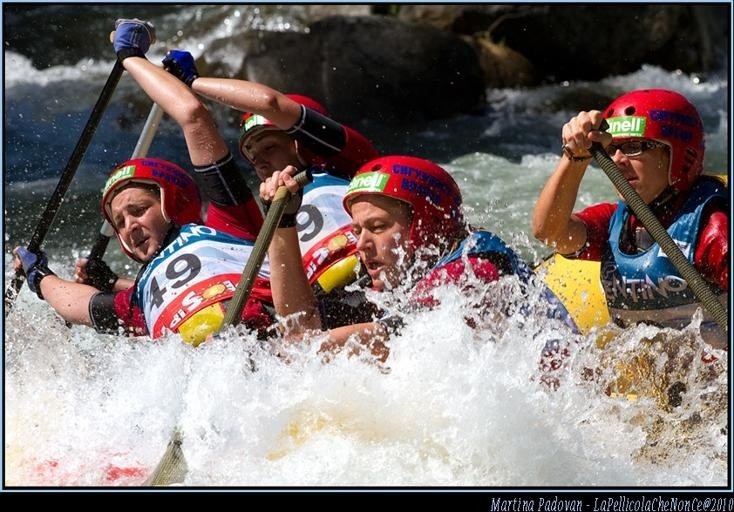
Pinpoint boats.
[2,162,728,486]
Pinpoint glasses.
[605,140,666,158]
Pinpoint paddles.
[141,168,314,487]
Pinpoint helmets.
[101,158,203,261]
[237,93,332,165]
[344,155,463,264]
[603,88,705,195]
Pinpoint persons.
[163,50,387,332]
[12,19,277,348]
[257,155,583,376]
[533,87,730,448]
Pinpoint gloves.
[162,50,201,86]
[83,258,118,292]
[113,17,160,60]
[15,247,53,296]
